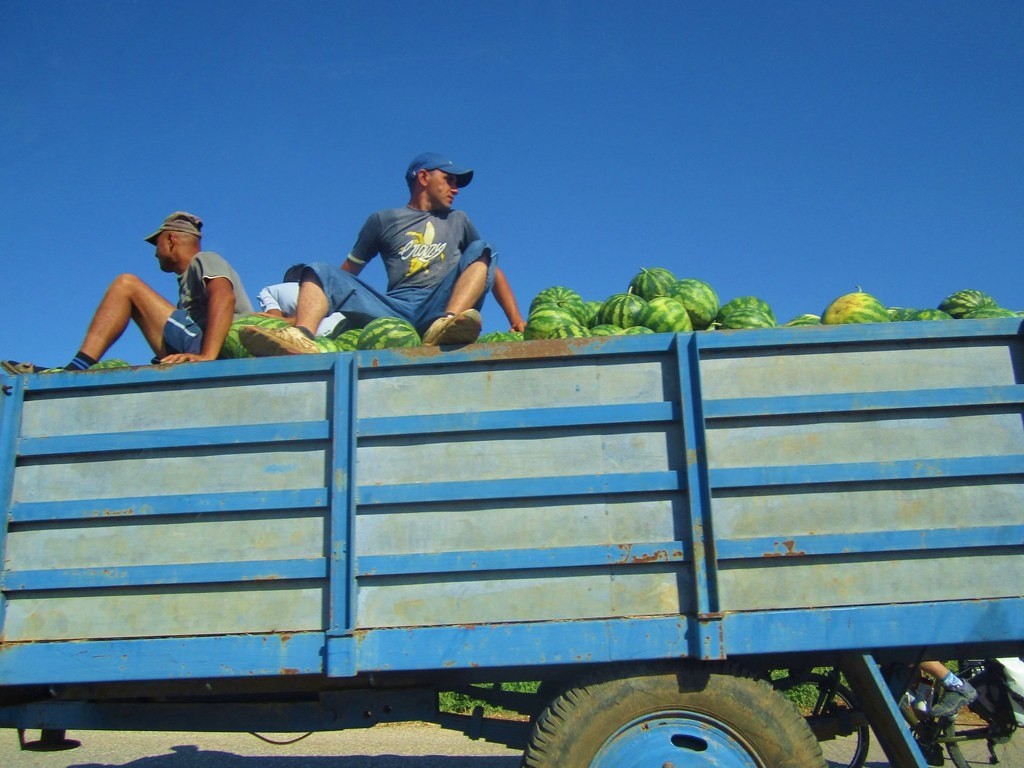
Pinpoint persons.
[0,209,255,376]
[256,262,345,337]
[236,151,530,359]
[901,659,979,767]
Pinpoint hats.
[144,211,204,246]
[405,152,473,188]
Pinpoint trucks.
[0,319,1024,768]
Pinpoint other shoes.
[0,361,36,375]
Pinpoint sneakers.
[422,308,482,347]
[931,678,979,718]
[238,325,321,357]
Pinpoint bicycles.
[771,653,1024,768]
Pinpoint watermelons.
[88,360,130,368]
[227,269,1023,367]
